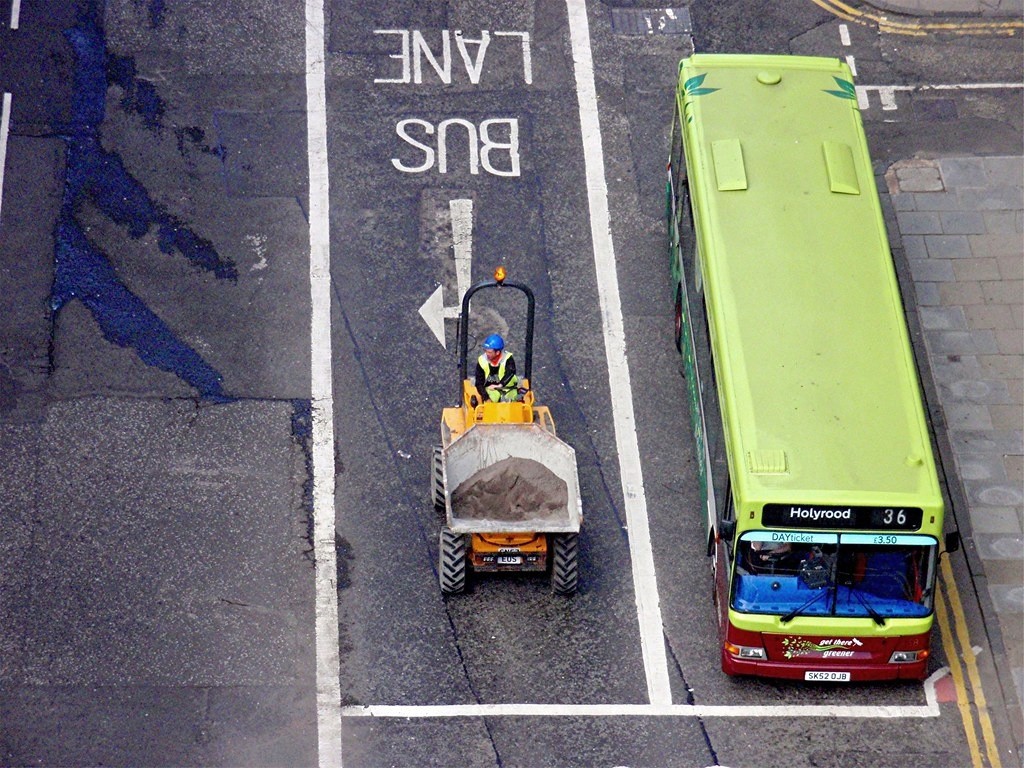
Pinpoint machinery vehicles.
[431,267,583,597]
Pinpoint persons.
[752,542,790,562]
[475,334,521,403]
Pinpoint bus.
[668,53,961,684]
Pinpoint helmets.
[481,335,504,350]
